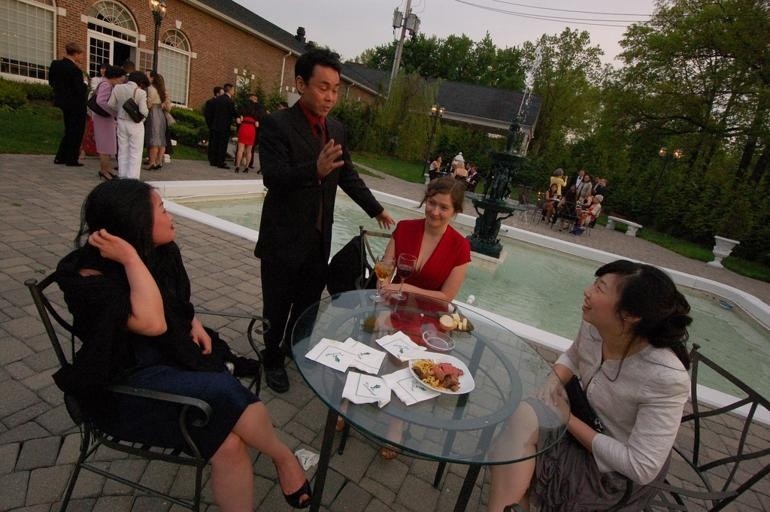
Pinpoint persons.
[487,259,691,510]
[336,176,470,461]
[255,47,395,393]
[55,179,313,511]
[49,42,290,182]
[506,114,525,152]
[429,154,607,233]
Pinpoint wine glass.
[390,253,417,301]
[367,253,397,303]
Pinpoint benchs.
[606,216,644,238]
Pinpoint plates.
[407,355,476,397]
[421,329,456,351]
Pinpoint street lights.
[420,101,448,179]
[645,142,688,218]
[143,0,167,75]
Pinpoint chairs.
[531,191,594,236]
[613,342,770,512]
[429,165,478,193]
[360,224,393,288]
[27,252,271,512]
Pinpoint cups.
[293,448,320,471]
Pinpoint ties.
[314,120,324,229]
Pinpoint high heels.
[242,168,248,173]
[143,162,163,171]
[235,167,240,173]
[99,168,120,181]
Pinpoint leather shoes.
[54,158,85,166]
[264,365,290,392]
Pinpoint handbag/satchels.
[163,108,176,127]
[564,375,606,433]
[327,235,367,296]
[122,98,144,123]
[88,93,112,118]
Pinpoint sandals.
[273,454,313,509]
[381,436,406,459]
